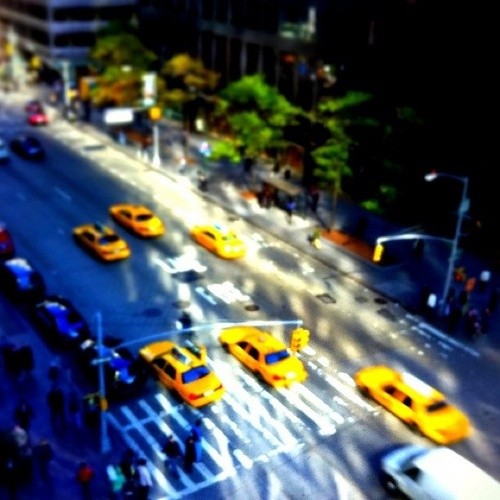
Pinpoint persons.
[1,310,205,500]
[48,81,320,226]
[416,239,499,344]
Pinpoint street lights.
[422,171,477,322]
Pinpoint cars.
[137,339,224,408]
[217,323,310,390]
[11,134,48,163]
[24,99,49,126]
[188,222,251,261]
[378,442,500,500]
[0,137,13,164]
[107,202,166,239]
[0,256,47,311]
[71,223,133,262]
[355,363,474,446]
[27,292,94,356]
[79,334,151,399]
[0,227,15,259]
